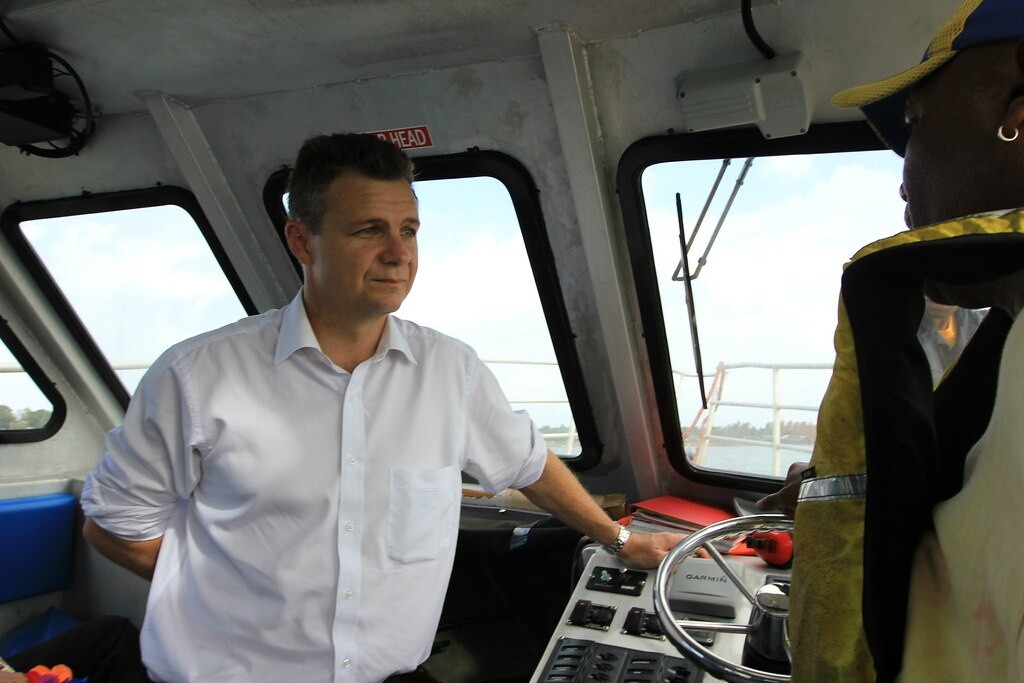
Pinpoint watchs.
[603,525,632,557]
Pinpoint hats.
[831,0,1024,158]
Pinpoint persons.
[81,134,716,683]
[788,0,1024,683]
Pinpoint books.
[629,494,739,531]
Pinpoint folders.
[630,493,748,543]
